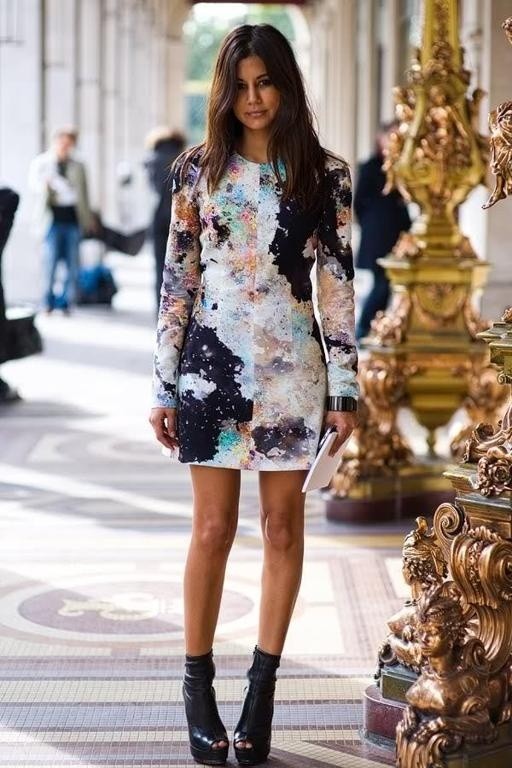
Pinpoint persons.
[24,125,188,322]
[352,119,410,342]
[150,24,360,768]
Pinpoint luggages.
[75,265,113,305]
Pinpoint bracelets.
[324,395,358,413]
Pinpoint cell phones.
[317,424,336,449]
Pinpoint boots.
[181,650,229,765]
[232,649,281,765]
